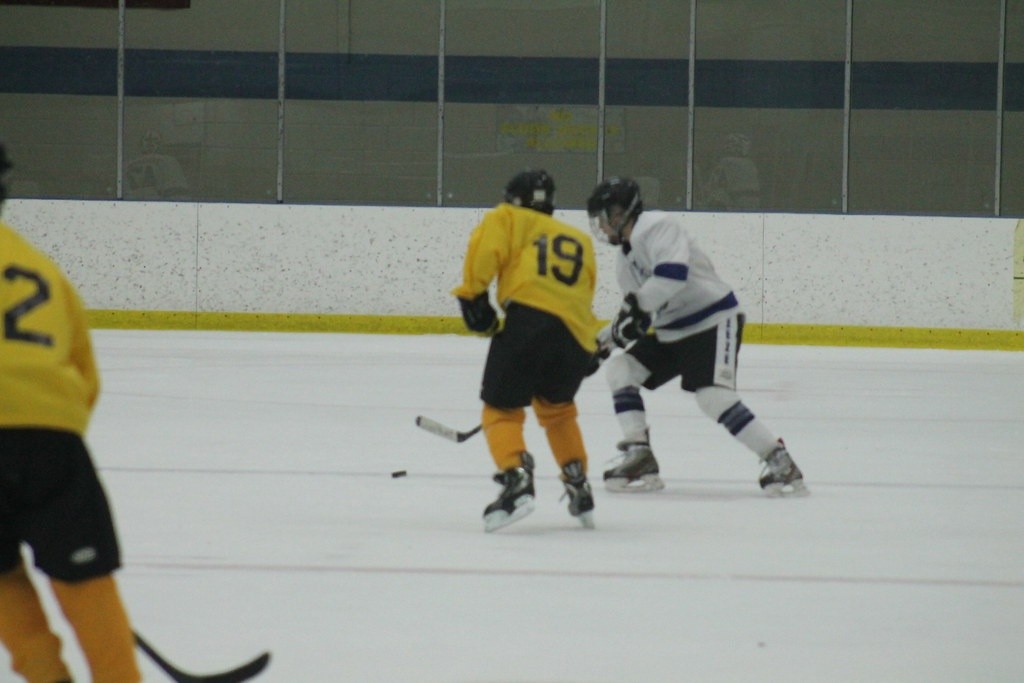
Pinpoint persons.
[586,175,811,498]
[0,142,141,683]
[451,169,599,532]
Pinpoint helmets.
[587,175,643,214]
[503,169,556,214]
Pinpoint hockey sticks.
[130,630,271,683]
[416,415,482,443]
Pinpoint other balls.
[390,470,408,479]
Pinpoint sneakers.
[483,451,535,531]
[758,438,809,497]
[559,458,596,529]
[603,426,664,494]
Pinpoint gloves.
[581,336,602,379]
[456,290,500,333]
[611,310,653,349]
